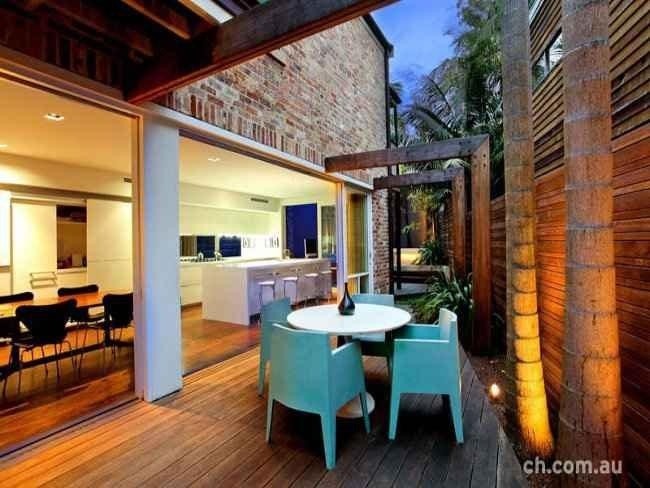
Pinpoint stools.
[256,270,333,308]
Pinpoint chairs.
[0,284,133,400]
[258,294,467,470]
